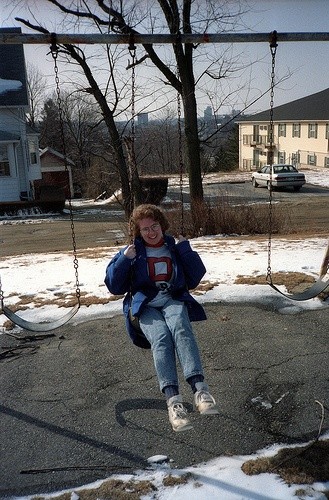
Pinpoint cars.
[250,163,307,193]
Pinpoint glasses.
[139,221,163,233]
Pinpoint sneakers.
[167,395,193,432]
[194,382,217,415]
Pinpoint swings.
[122,42,184,350]
[266,41,329,301]
[0,44,81,332]
